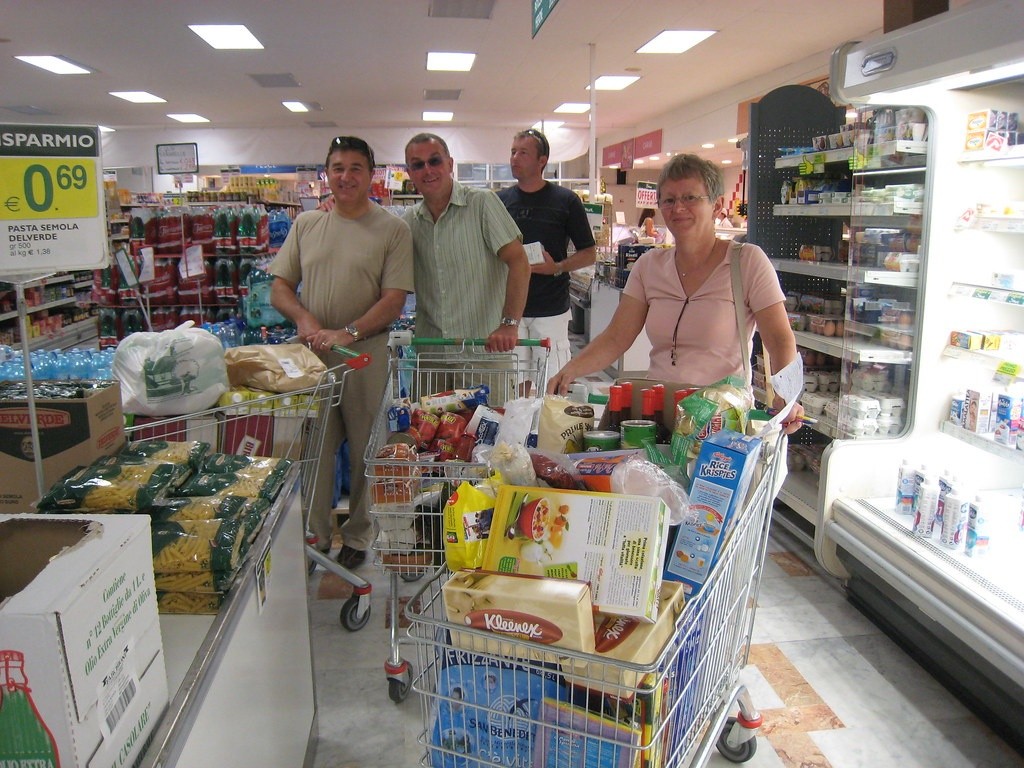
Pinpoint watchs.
[553,263,562,277]
[345,324,359,343]
[500,316,519,327]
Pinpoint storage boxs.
[0,381,126,515]
[1,507,171,768]
[443,428,760,701]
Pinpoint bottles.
[381,316,417,390]
[102,201,310,358]
[605,383,703,448]
[0,344,125,393]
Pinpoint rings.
[322,342,325,345]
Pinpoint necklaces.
[682,273,686,277]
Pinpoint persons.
[267,137,415,571]
[546,153,805,434]
[638,209,658,256]
[493,128,597,389]
[717,208,733,239]
[315,133,531,408]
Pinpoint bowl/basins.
[829,107,929,150]
[519,497,550,542]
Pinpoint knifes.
[504,492,518,536]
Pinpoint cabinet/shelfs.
[747,138,926,551]
[1,178,589,353]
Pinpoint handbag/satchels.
[748,419,789,507]
[112,318,229,417]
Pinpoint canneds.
[185,191,247,203]
[453,433,477,462]
[387,408,467,476]
[582,420,658,453]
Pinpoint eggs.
[800,351,844,366]
[788,444,825,474]
[801,311,913,348]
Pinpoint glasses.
[408,156,446,170]
[526,129,543,146]
[331,136,367,150]
[656,195,708,210]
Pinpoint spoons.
[508,493,529,540]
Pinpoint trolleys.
[115,334,379,632]
[365,335,553,699]
[383,403,766,767]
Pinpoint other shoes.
[308,549,329,575]
[337,546,363,570]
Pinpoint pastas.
[82,441,280,615]
